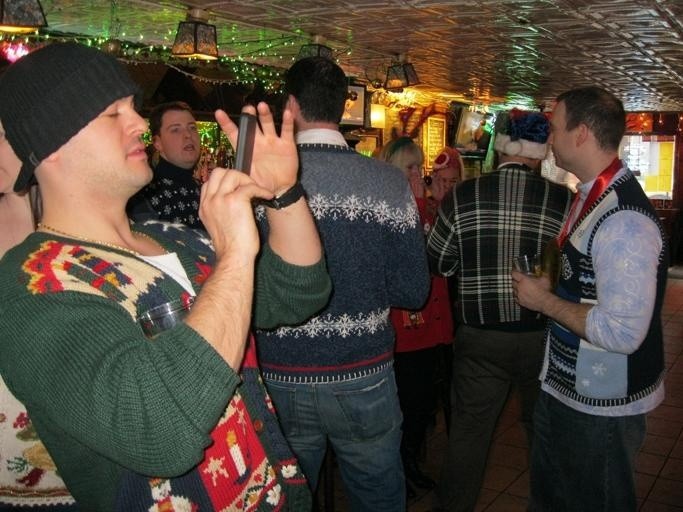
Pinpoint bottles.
[216,146,226,169]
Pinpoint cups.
[511,254,544,277]
[141,297,196,336]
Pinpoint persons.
[1,43,577,511]
[511,87,668,512]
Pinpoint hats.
[0,43,145,192]
[432,147,463,177]
[494,108,552,160]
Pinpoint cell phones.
[234,113,257,177]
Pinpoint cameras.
[423,176,432,186]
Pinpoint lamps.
[0,0,45,35]
[294,35,333,63]
[383,53,420,90]
[169,5,220,61]
